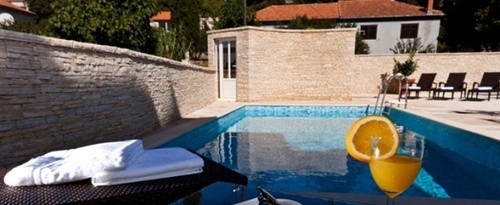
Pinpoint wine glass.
[370,134,425,205]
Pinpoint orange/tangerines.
[346,114,400,163]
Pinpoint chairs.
[400,72,438,99]
[467,71,500,101]
[434,72,468,101]
[1,149,249,205]
